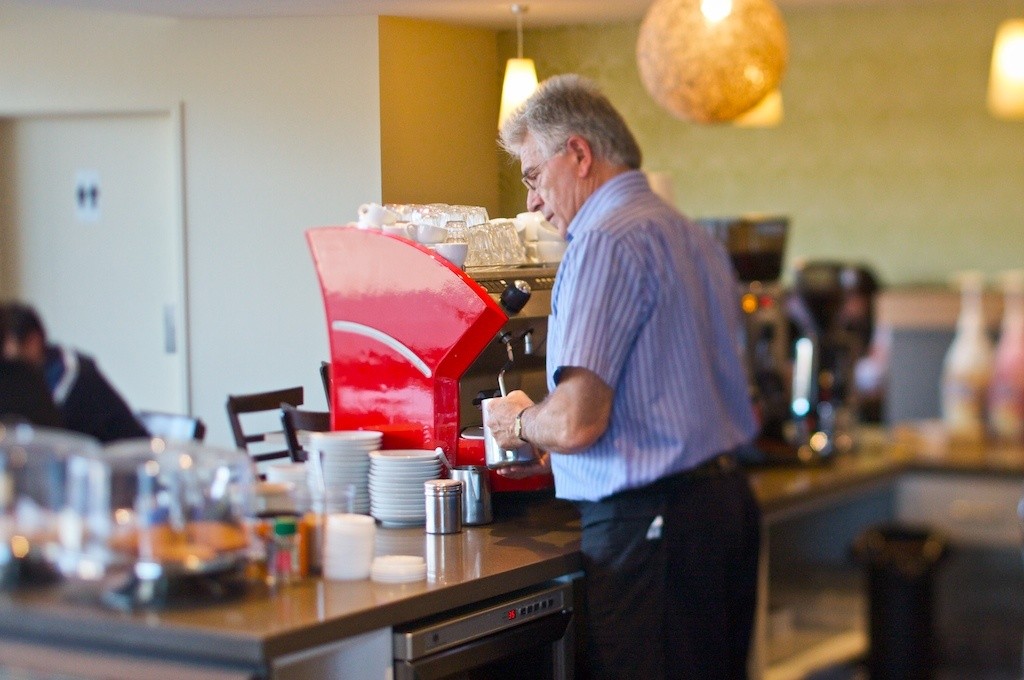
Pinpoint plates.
[307,431,445,529]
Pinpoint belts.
[657,451,735,488]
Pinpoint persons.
[0,301,151,446]
[486,76,764,680]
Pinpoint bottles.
[985,268,1024,443]
[245,513,319,598]
[425,478,463,534]
[939,271,990,441]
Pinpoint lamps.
[634,0,791,129]
[497,4,539,133]
[986,17,1024,124]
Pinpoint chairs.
[226,361,330,479]
[136,408,207,444]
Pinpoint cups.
[323,512,376,580]
[448,465,493,525]
[348,203,566,270]
[481,397,537,471]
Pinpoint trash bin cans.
[849,521,943,680]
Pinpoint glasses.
[521,139,591,192]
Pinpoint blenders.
[690,213,838,470]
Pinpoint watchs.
[515,407,529,443]
